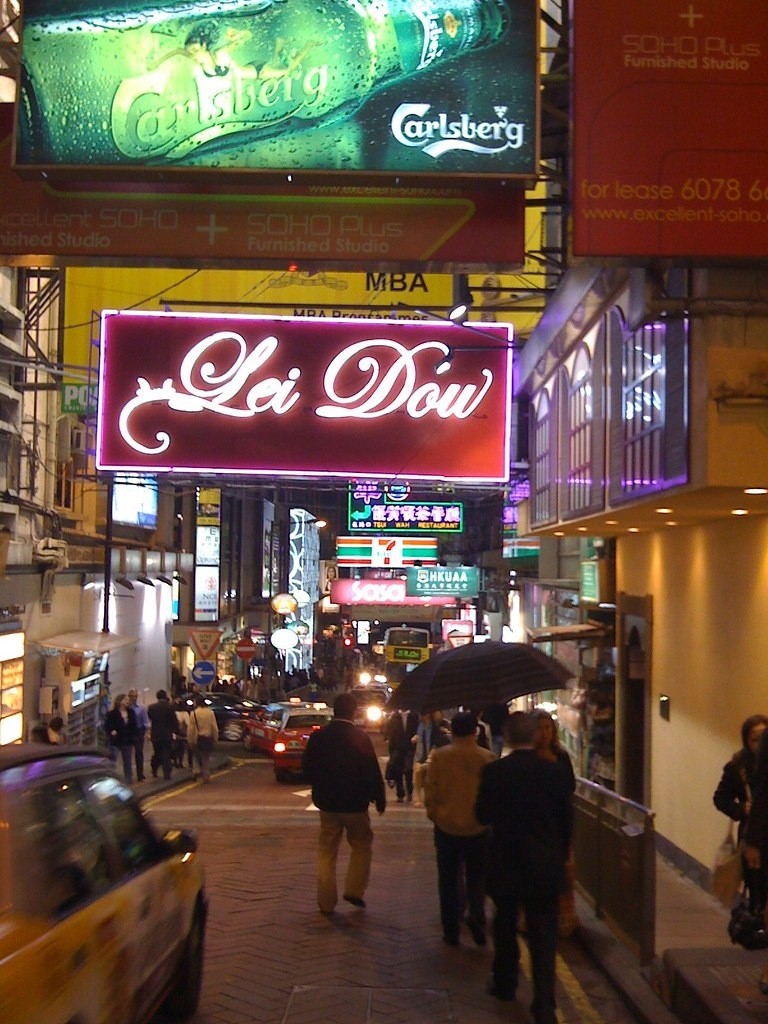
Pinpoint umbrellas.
[383,636,577,710]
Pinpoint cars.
[274,702,339,782]
[245,698,314,756]
[1,747,209,1024]
[345,680,396,733]
[177,693,269,744]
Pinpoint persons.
[422,709,576,1023]
[301,695,387,914]
[387,702,509,804]
[47,717,64,744]
[173,620,382,703]
[103,686,219,783]
[322,567,336,594]
[711,714,767,994]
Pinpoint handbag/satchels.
[711,834,743,907]
[385,751,408,781]
[727,865,768,951]
[196,734,214,754]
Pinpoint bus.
[383,626,430,673]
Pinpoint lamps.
[449,285,555,322]
[172,575,187,584]
[136,570,155,587]
[156,575,172,586]
[79,572,95,590]
[413,559,423,569]
[115,573,135,590]
[435,344,521,374]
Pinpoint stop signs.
[237,639,256,661]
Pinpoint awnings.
[524,619,613,642]
[38,629,141,657]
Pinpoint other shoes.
[137,775,146,782]
[531,1009,558,1024]
[344,894,367,907]
[473,937,487,946]
[191,772,200,782]
[406,789,413,802]
[486,971,516,1001]
[396,798,404,803]
[559,926,596,953]
[759,969,768,995]
[173,763,186,769]
[441,932,459,947]
